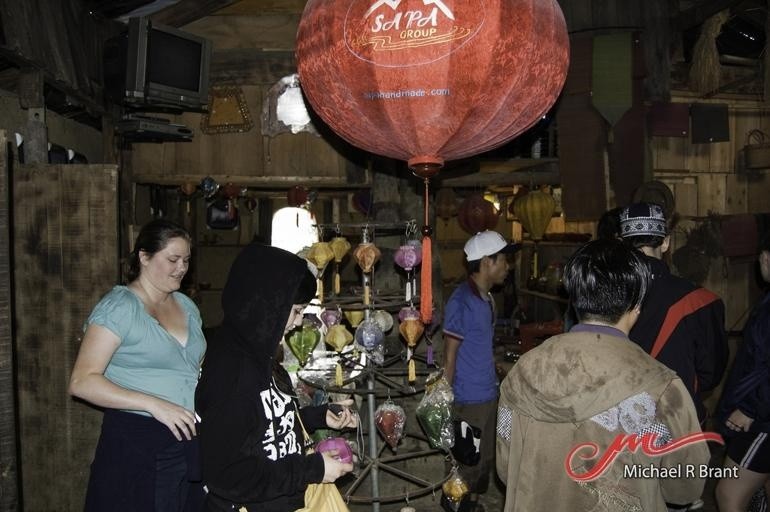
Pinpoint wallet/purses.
[315,436,352,464]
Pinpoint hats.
[631,180,675,222]
[617,203,667,238]
[463,229,524,262]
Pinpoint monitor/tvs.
[689,103,731,145]
[126,17,211,105]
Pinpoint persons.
[558,209,622,334]
[715,249,769,512]
[617,198,731,511]
[66,216,207,510]
[439,228,511,511]
[189,242,355,511]
[495,238,710,512]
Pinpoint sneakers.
[440,497,487,512]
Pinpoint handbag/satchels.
[449,418,482,468]
[235,435,349,512]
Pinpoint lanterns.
[281,231,451,464]
[514,179,557,277]
[294,1,570,324]
[460,191,500,232]
[179,176,243,214]
[436,189,457,226]
